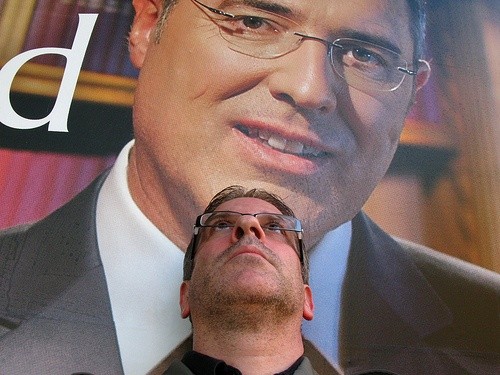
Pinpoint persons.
[158,184,325,374]
[1,0,499,374]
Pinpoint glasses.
[194,211,304,266]
[192,0,418,96]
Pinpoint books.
[0,0,153,81]
